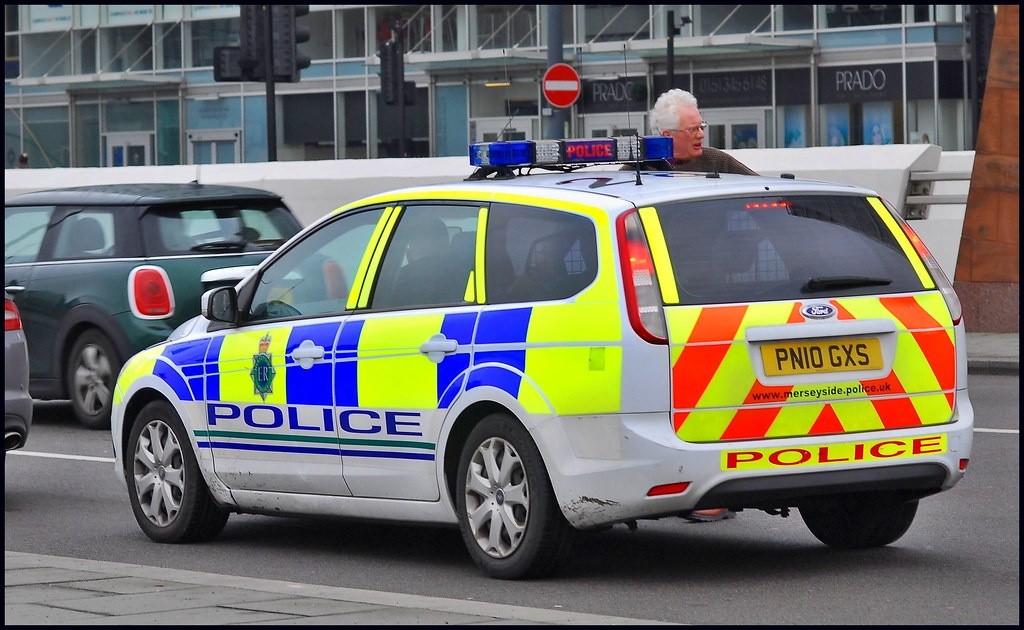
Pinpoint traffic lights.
[375,44,397,101]
[272,3,312,84]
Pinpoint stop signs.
[539,61,582,110]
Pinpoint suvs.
[6,184,352,432]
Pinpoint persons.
[396,215,449,301]
[618,88,771,289]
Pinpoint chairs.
[443,231,517,297]
[74,218,105,250]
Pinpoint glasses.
[669,121,708,135]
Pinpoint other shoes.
[688,508,737,522]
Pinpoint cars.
[109,135,975,584]
[3,297,36,448]
